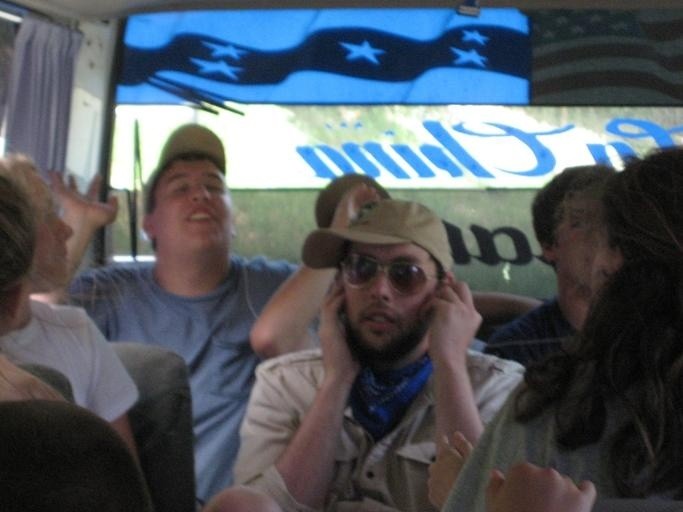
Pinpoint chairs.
[0,340,195,512]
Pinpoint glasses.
[340,252,440,295]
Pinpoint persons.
[1,122,683,512]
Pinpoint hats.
[142,122,225,213]
[302,198,454,273]
[315,174,391,227]
